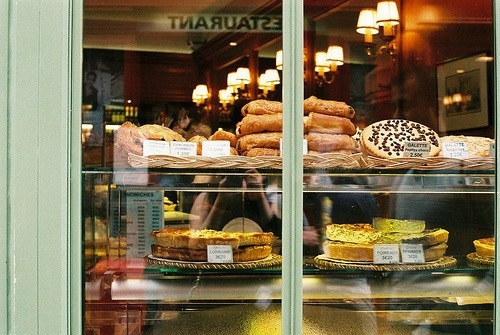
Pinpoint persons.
[387,65,495,335]
[188,164,377,335]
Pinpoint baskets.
[127,153,361,169]
[364,156,496,171]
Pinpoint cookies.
[360,118,441,158]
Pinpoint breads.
[437,135,493,156]
[473,238,494,260]
[116,96,358,156]
[151,227,273,261]
[322,216,450,262]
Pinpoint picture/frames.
[433,47,492,135]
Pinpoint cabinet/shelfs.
[80,169,497,335]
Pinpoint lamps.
[226,66,251,93]
[275,49,283,70]
[356,0,400,65]
[258,68,281,100]
[191,84,209,108]
[218,88,235,112]
[313,45,345,87]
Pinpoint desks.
[163,210,200,229]
